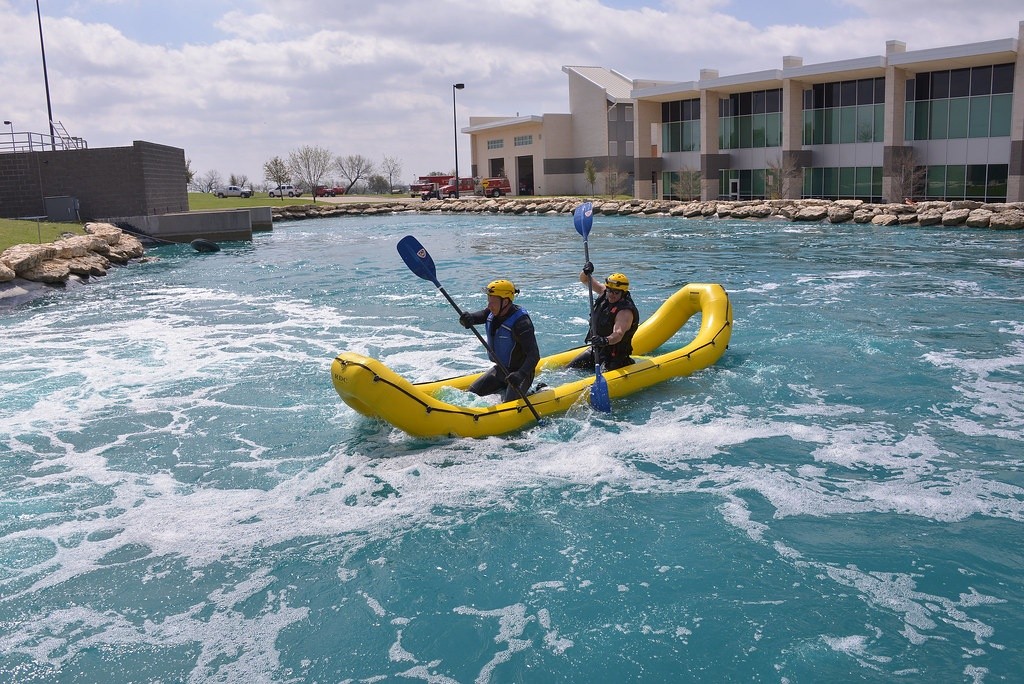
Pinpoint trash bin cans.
[44,197,81,223]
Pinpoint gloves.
[505,371,525,390]
[591,335,609,347]
[460,311,474,329]
[582,261,594,276]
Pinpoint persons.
[563,261,639,371]
[459,280,540,404]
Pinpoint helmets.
[606,273,629,291]
[485,280,515,303]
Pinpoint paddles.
[397,235,542,424]
[573,202,611,412]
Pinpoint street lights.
[452,83,465,198]
[4,120,16,152]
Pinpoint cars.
[268,184,303,198]
[391,189,402,194]
[333,183,346,195]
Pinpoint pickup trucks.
[215,185,253,198]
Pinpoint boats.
[331,281,734,441]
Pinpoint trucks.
[409,176,456,199]
[419,182,443,201]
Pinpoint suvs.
[313,184,336,197]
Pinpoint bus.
[436,176,512,200]
